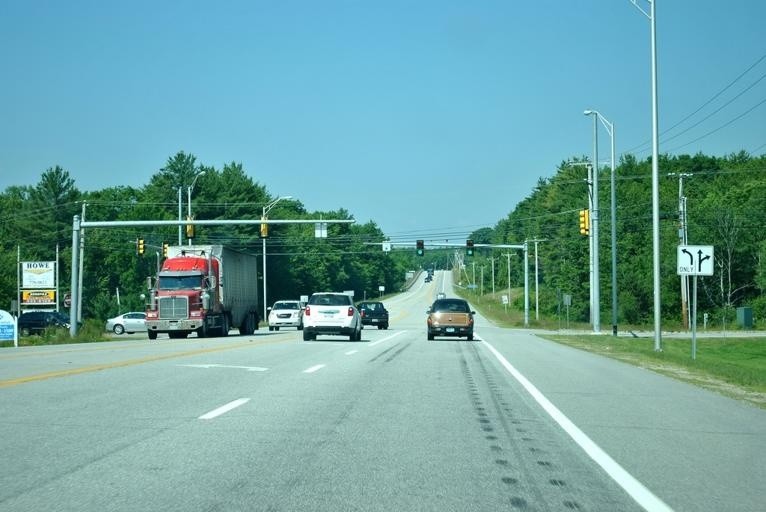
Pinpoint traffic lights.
[579,210,590,238]
[417,240,424,257]
[135,237,145,256]
[161,241,168,258]
[467,239,474,257]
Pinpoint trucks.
[140,243,261,340]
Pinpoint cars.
[437,293,446,299]
[423,263,434,283]
[105,312,148,335]
[356,301,389,329]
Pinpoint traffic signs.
[676,244,715,277]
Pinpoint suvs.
[425,297,476,342]
[266,299,305,331]
[18,311,83,337]
[300,292,362,341]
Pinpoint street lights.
[171,186,182,244]
[585,110,618,337]
[262,195,293,323]
[668,172,697,326]
[188,171,208,244]
